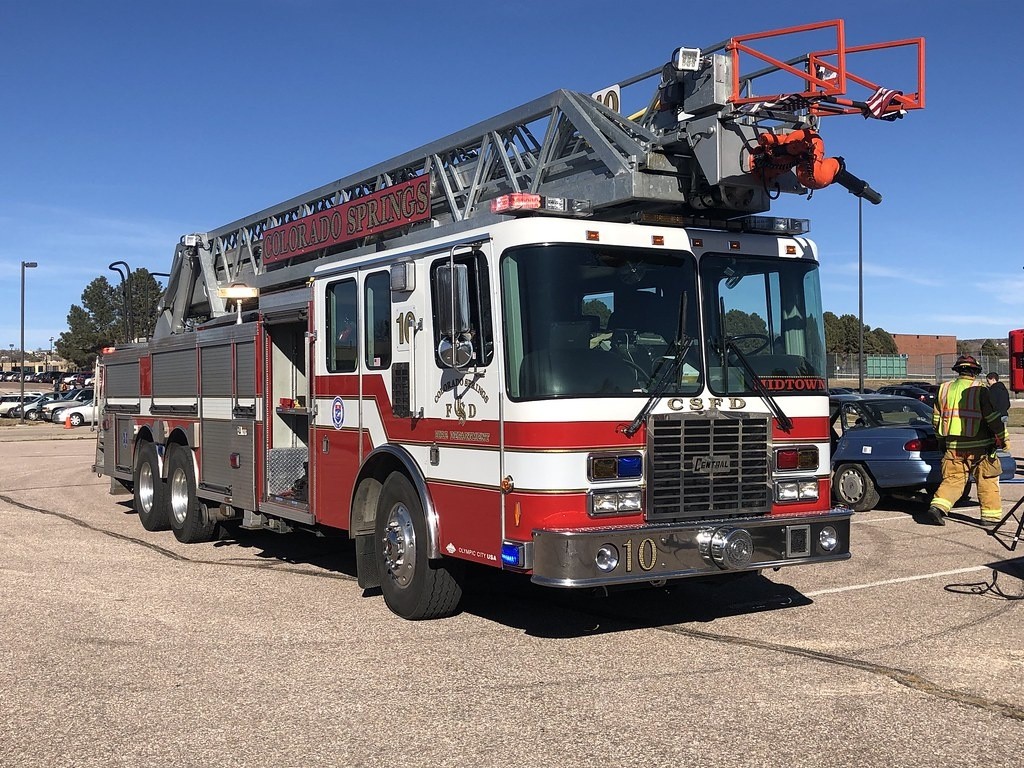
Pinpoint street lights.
[9,344,14,371]
[20,261,38,424]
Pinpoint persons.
[983,371,1012,451]
[52,378,85,400]
[928,356,1008,526]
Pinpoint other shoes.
[928,507,945,526]
[982,520,1006,526]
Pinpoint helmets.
[952,356,982,373]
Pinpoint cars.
[828,382,940,409]
[0,371,95,386]
[0,388,98,426]
[829,395,1017,512]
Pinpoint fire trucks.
[90,19,925,620]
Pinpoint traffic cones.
[64,408,74,429]
